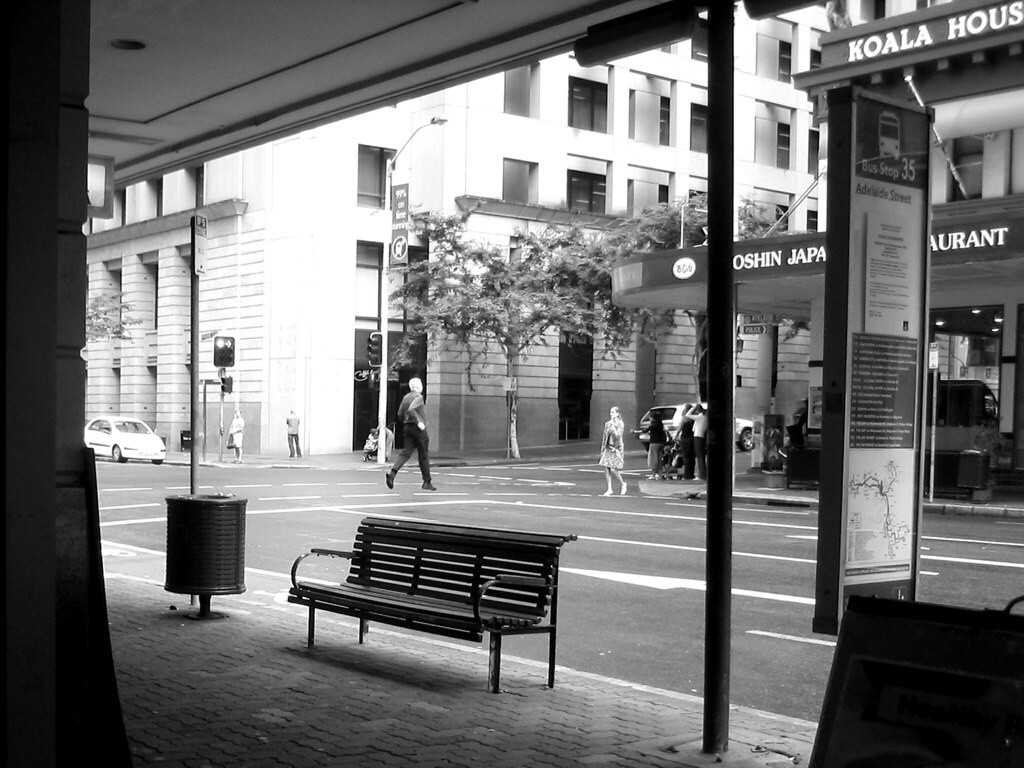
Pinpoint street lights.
[376,116,452,467]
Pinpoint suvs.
[638,401,755,452]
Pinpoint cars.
[84,415,166,465]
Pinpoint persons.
[228,409,245,464]
[599,406,627,495]
[643,411,672,480]
[386,378,437,490]
[368,425,394,463]
[286,410,302,458]
[674,403,708,480]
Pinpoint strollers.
[655,434,685,481]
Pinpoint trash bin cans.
[180,429,192,452]
[956,448,990,489]
[163,493,247,621]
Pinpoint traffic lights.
[366,331,384,368]
[212,336,236,367]
[221,376,233,393]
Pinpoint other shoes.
[604,490,613,496]
[693,477,704,480]
[645,474,652,477]
[386,473,394,489]
[621,482,628,495]
[422,481,437,491]
[647,476,656,480]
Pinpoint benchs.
[288,517,576,693]
